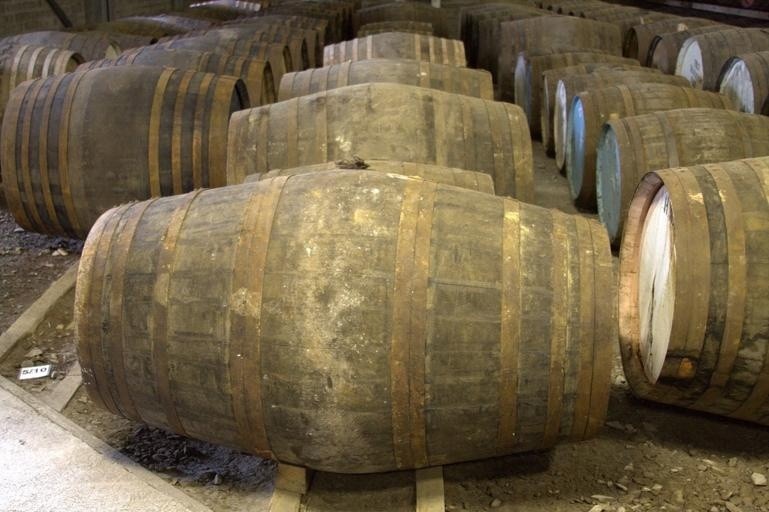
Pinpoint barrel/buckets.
[619,156,769,426]
[1,4,768,209]
[73,169,616,473]
